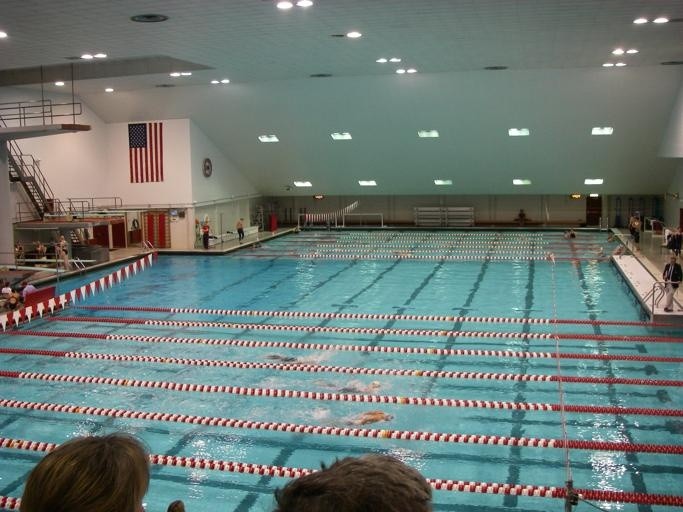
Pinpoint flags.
[127,122,163,183]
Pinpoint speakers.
[179,212,185,217]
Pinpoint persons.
[662,256,683,312]
[564,229,576,240]
[236,218,244,244]
[631,216,642,250]
[612,242,626,259]
[18,430,150,512]
[166,499,185,512]
[255,205,265,232]
[270,452,433,512]
[518,208,525,227]
[202,221,209,249]
[629,212,636,239]
[0,232,71,311]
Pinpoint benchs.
[23,285,56,307]
